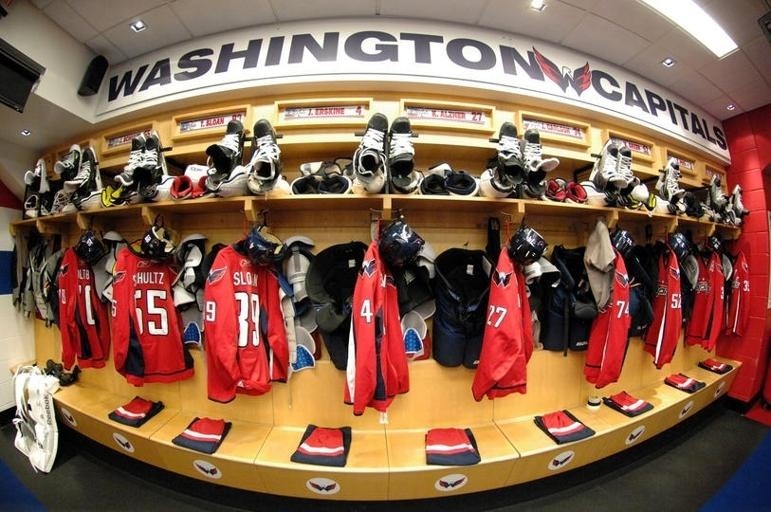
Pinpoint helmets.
[74,230,107,266]
[670,232,693,264]
[610,230,634,258]
[247,226,286,267]
[513,226,546,266]
[143,225,181,262]
[380,217,424,268]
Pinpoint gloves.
[158,171,207,199]
[545,175,588,204]
[291,159,352,197]
[395,169,480,198]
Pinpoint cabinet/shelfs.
[9,91,742,512]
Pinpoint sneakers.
[389,116,416,180]
[25,145,101,218]
[659,161,686,203]
[112,132,164,200]
[207,120,245,180]
[356,113,387,178]
[250,118,282,184]
[497,121,558,199]
[712,173,751,225]
[590,140,642,200]
